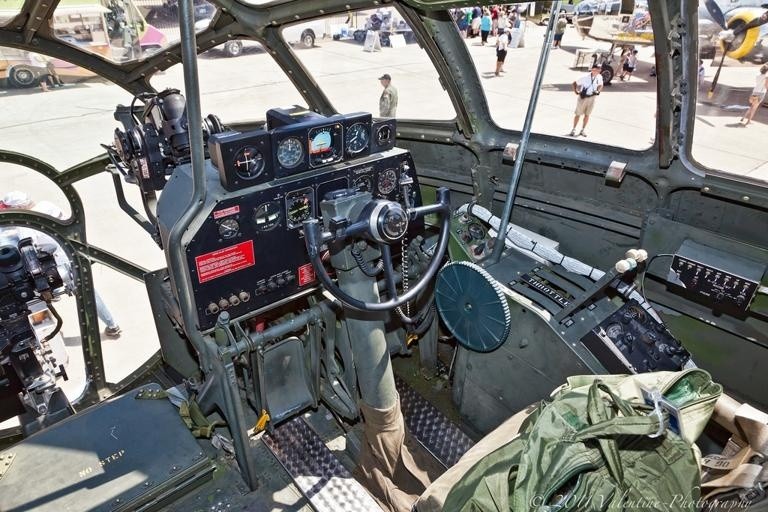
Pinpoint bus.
[0,0,170,90]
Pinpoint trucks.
[193,6,327,57]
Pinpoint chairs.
[412,368,767,511]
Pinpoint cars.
[157,1,214,20]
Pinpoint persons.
[446,4,519,45]
[377,71,403,118]
[698,59,707,86]
[621,49,637,80]
[28,52,52,92]
[492,28,510,78]
[613,46,629,77]
[553,13,567,47]
[92,288,122,338]
[570,59,605,137]
[738,65,768,123]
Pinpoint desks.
[572,48,602,70]
[379,27,411,43]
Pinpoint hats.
[379,74,391,80]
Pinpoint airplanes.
[572,0,768,100]
